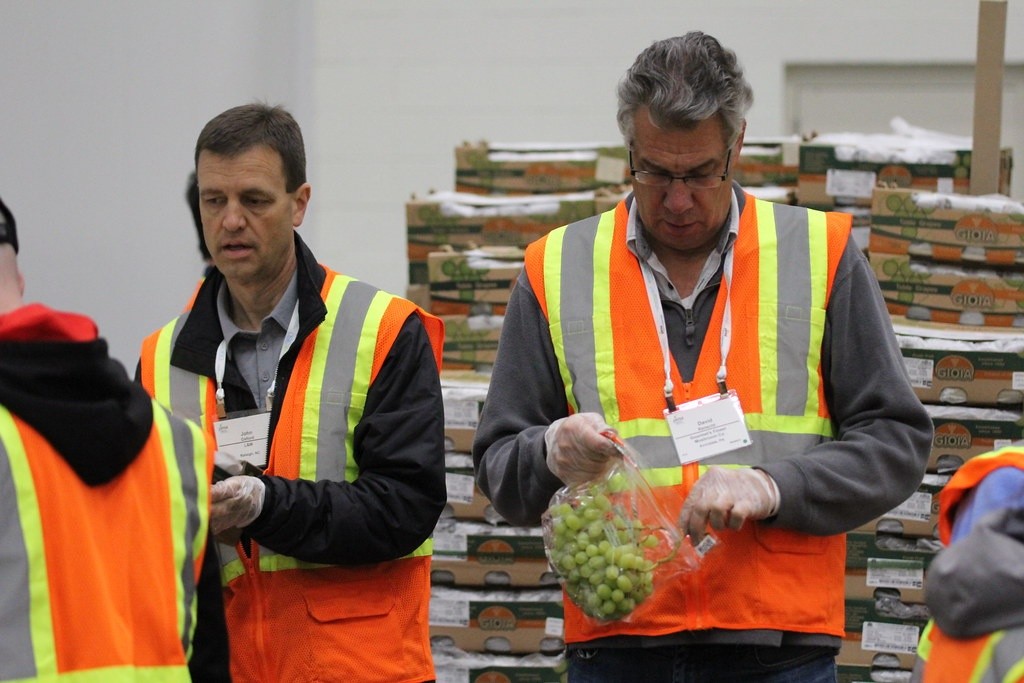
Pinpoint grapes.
[547,474,681,621]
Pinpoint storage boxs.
[402,133,1024,683]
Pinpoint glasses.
[630,143,732,189]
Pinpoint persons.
[134,102,448,683]
[1,198,214,683]
[469,31,935,683]
[911,440,1024,683]
